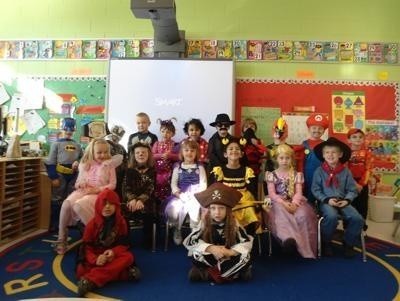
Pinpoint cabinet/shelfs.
[0,156,50,261]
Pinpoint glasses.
[217,123,229,127]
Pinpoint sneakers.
[56,243,65,254]
[128,264,141,281]
[78,276,93,298]
[339,244,355,257]
[282,238,296,256]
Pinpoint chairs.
[75,177,369,264]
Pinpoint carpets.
[0,222,400,301]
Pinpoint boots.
[187,264,209,282]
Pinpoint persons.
[167,112,264,245]
[182,181,254,284]
[41,110,180,254]
[75,187,143,298]
[263,114,372,261]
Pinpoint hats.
[306,113,329,130]
[194,182,243,208]
[314,136,351,162]
[209,114,236,127]
